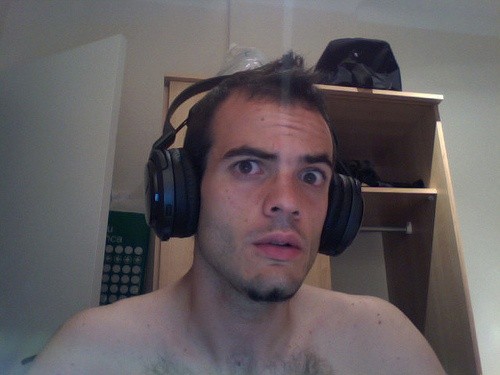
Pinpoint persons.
[27,50,448,375]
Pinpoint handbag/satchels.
[314,38,402,91]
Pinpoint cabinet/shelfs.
[152,73,483,375]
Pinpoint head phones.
[143,75,364,256]
